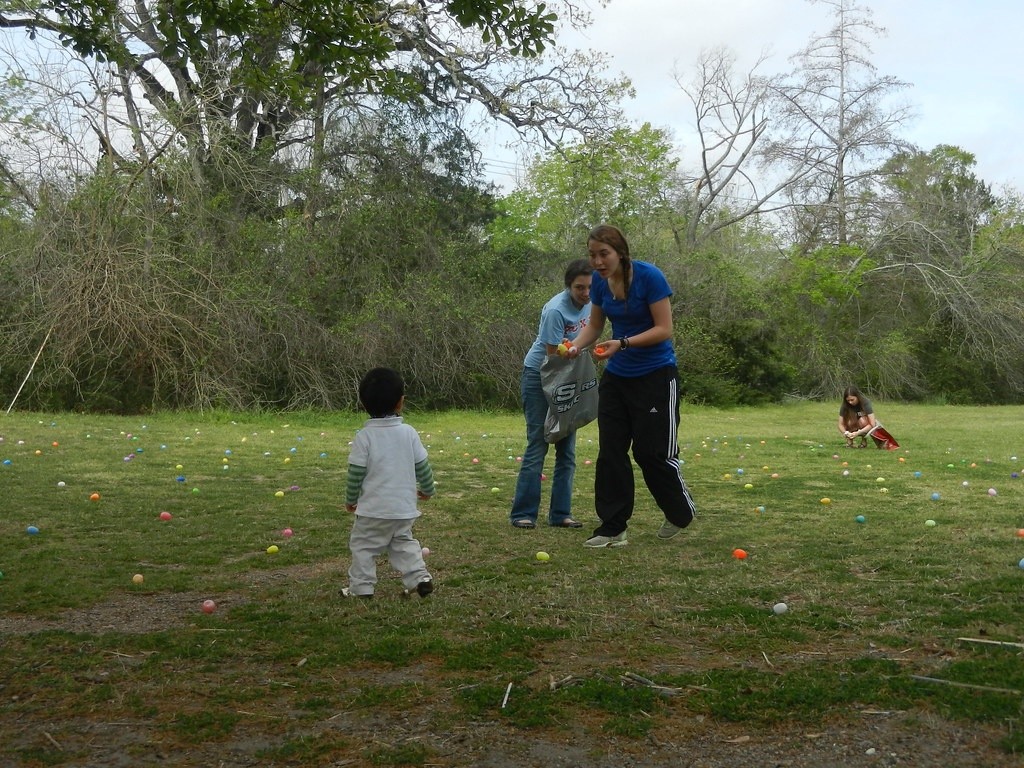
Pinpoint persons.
[838,386,876,449]
[510,259,598,529]
[339,367,438,600]
[555,225,696,547]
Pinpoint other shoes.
[513,520,534,528]
[846,442,854,447]
[339,587,376,599]
[858,443,867,448]
[403,581,433,598]
[549,521,583,528]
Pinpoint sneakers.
[656,519,682,540]
[583,530,627,548]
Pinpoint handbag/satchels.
[539,350,600,444]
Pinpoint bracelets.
[624,337,630,348]
[618,335,627,352]
